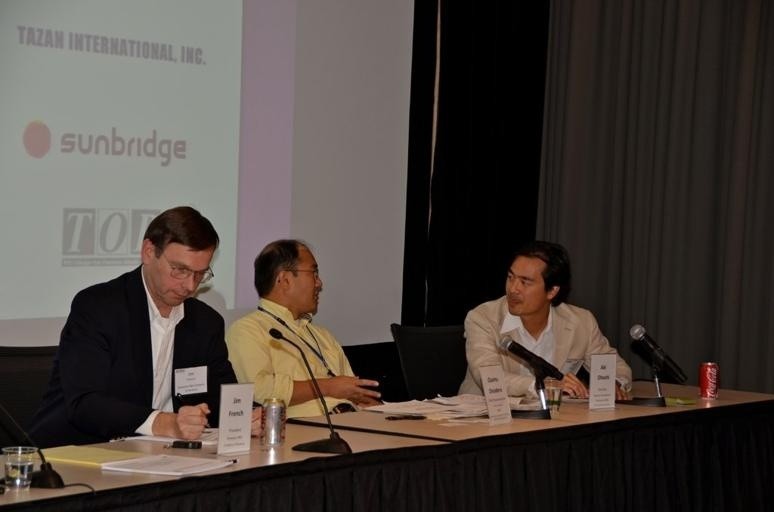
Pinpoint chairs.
[1,346,61,450]
[389,322,465,399]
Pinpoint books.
[100,454,233,475]
[361,392,544,424]
[26,444,148,467]
[123,425,221,447]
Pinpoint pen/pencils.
[385,416,426,420]
[177,393,211,428]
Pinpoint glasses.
[163,253,214,283]
[287,270,318,279]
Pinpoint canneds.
[699,362,720,401]
[261,399,286,447]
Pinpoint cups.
[542,378,562,412]
[1,445,38,490]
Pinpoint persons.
[223,238,385,419]
[29,205,265,440]
[457,240,634,401]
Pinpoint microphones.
[268,327,352,454]
[499,335,564,381]
[0,403,64,489]
[628,323,688,383]
